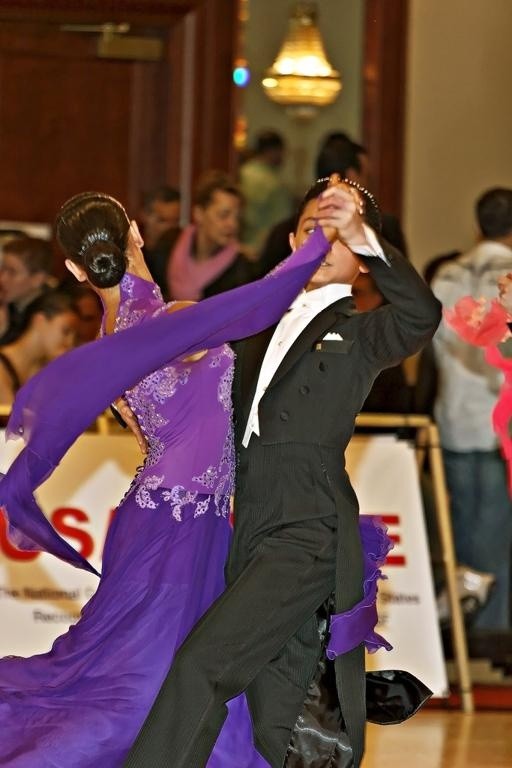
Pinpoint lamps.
[261,10,343,119]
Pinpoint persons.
[1,129,511,768]
